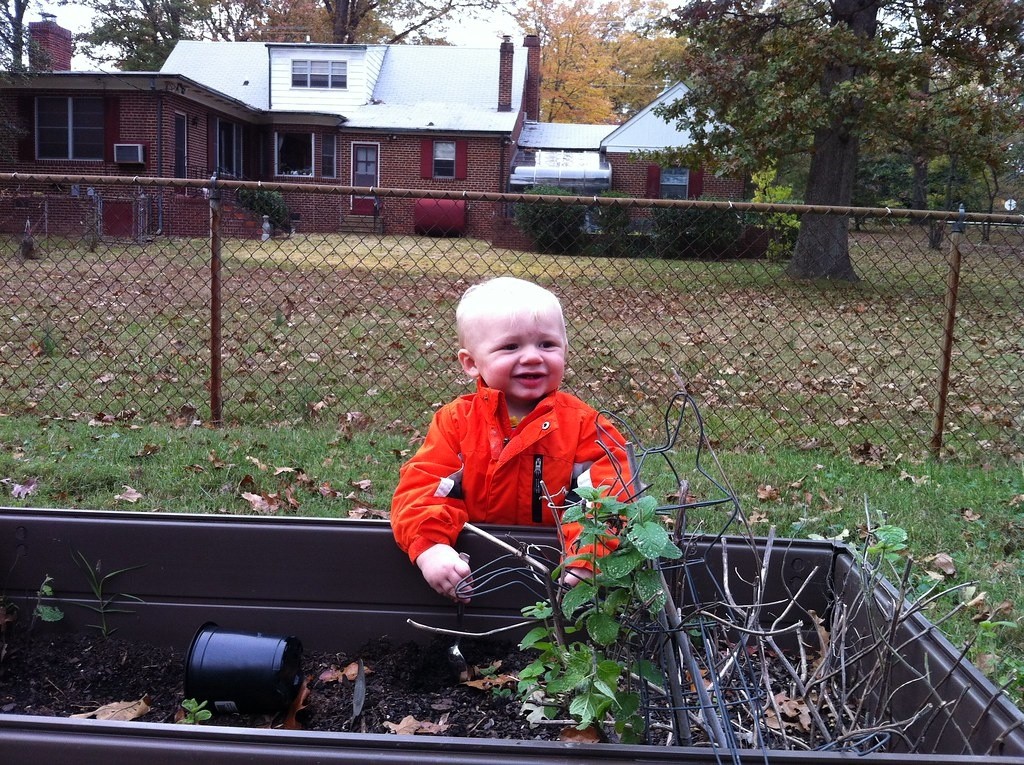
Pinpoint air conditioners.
[114,143,146,166]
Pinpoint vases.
[180,619,302,712]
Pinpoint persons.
[390,276,636,604]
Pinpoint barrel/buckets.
[184,621,304,707]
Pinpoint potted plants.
[0,503,1024,765]
[223,186,290,239]
[290,211,302,234]
